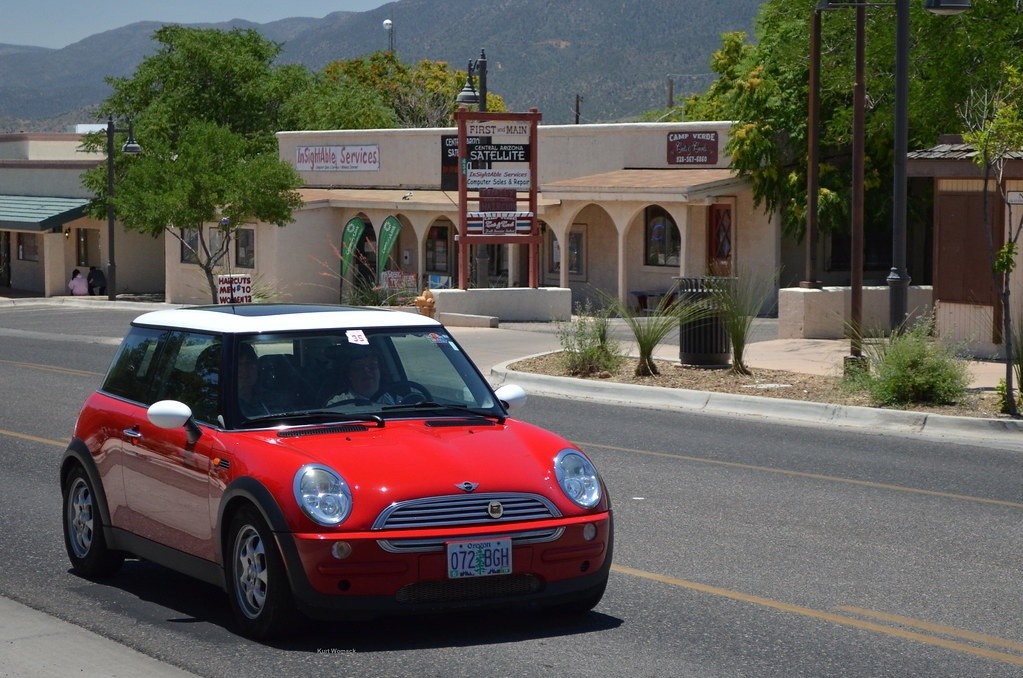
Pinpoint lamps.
[65,228,71,239]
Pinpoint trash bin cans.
[671,276,739,366]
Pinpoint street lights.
[104,112,142,300]
[456,47,491,289]
[884,0,972,338]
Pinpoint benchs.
[631,289,678,317]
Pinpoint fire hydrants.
[413,289,437,319]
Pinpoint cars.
[57,301,615,644]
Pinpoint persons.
[87,266,106,295]
[68,269,89,295]
[326,343,396,404]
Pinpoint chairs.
[257,354,310,418]
